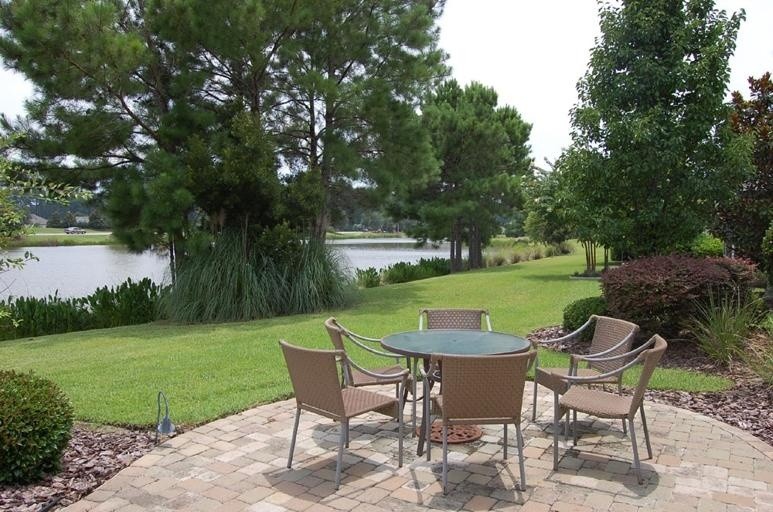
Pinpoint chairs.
[426,350,538,495]
[419,307,492,394]
[551,333,668,485]
[278,340,410,490]
[529,314,640,441]
[326,317,414,422]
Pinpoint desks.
[381,328,530,457]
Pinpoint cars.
[64,227,86,235]
